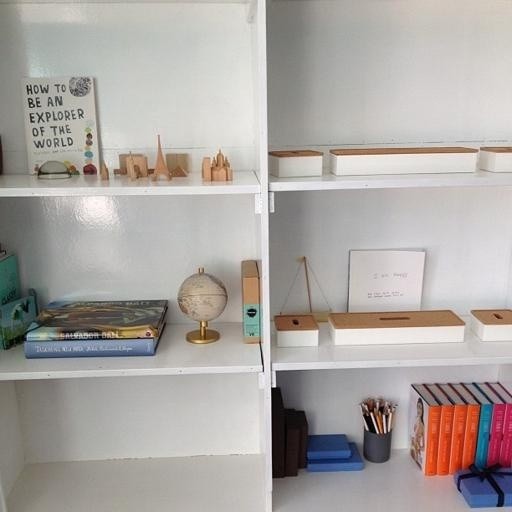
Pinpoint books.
[22,339,158,357]
[23,300,173,341]
[0,250,37,350]
[408,382,511,475]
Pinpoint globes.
[178,267,228,344]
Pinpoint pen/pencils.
[359,398,398,434]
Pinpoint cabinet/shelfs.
[0,0,512,512]
